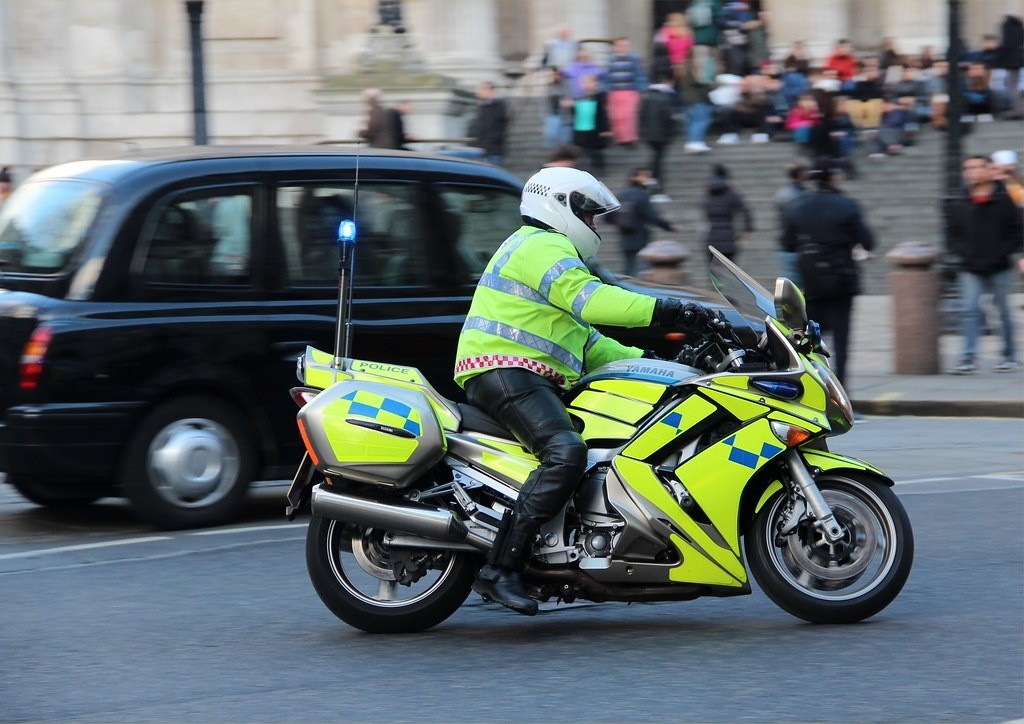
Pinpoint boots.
[470,508,540,616]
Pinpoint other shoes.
[950,361,981,374]
[751,133,768,142]
[994,362,1018,372]
[683,140,708,152]
[716,134,739,145]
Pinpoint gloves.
[654,296,710,332]
[653,341,682,360]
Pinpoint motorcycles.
[288,247,915,635]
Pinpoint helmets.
[520,166,622,263]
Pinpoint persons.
[0,165,10,202]
[350,0,1024,392]
[453,168,723,612]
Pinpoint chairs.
[382,213,468,283]
[298,196,378,283]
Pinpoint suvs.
[0,147,776,529]
